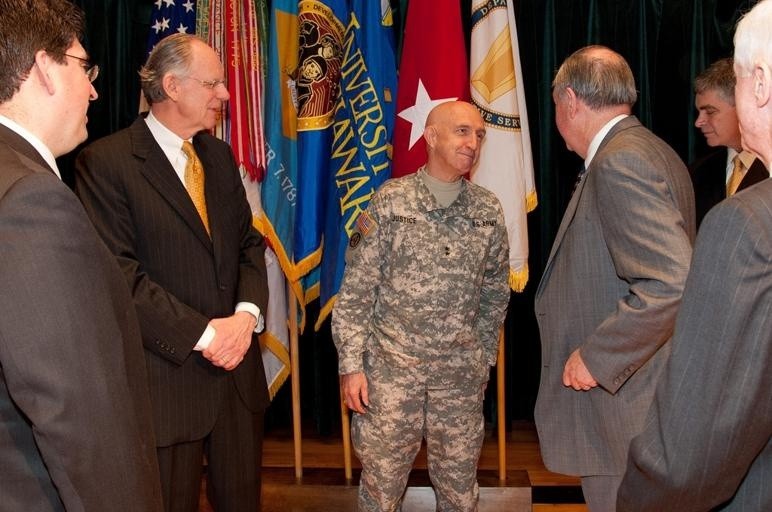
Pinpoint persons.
[531,43,698,512]
[0,1,167,512]
[615,0,771,512]
[680,54,770,239]
[61,31,271,512]
[330,98,510,510]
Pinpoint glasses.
[178,77,227,89]
[45,48,99,82]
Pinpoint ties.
[570,164,586,197]
[726,156,742,194]
[181,140,212,241]
